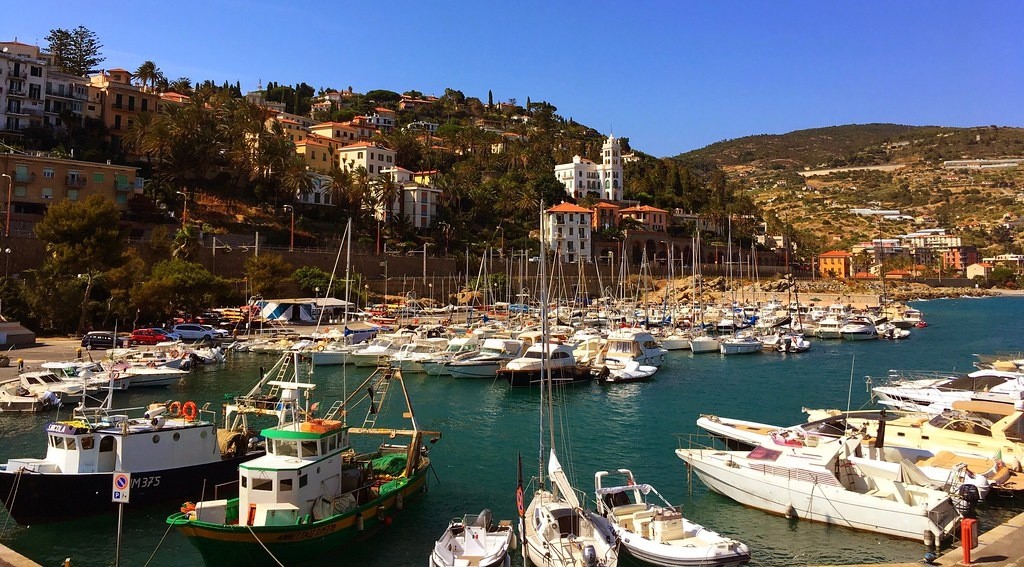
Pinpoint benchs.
[614,503,648,530]
[632,511,653,538]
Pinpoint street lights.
[177,191,186,228]
[371,214,380,255]
[284,205,294,251]
[1,173,13,238]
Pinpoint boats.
[594,469,751,566]
[429,509,520,567]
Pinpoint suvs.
[171,324,219,341]
[82,332,132,350]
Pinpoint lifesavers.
[109,370,120,379]
[182,401,197,420]
[170,350,179,358]
[169,401,183,418]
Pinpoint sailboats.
[0,198,1024,567]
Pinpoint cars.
[145,328,183,343]
[131,329,168,345]
[201,324,229,338]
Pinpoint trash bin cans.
[961,518,978,564]
